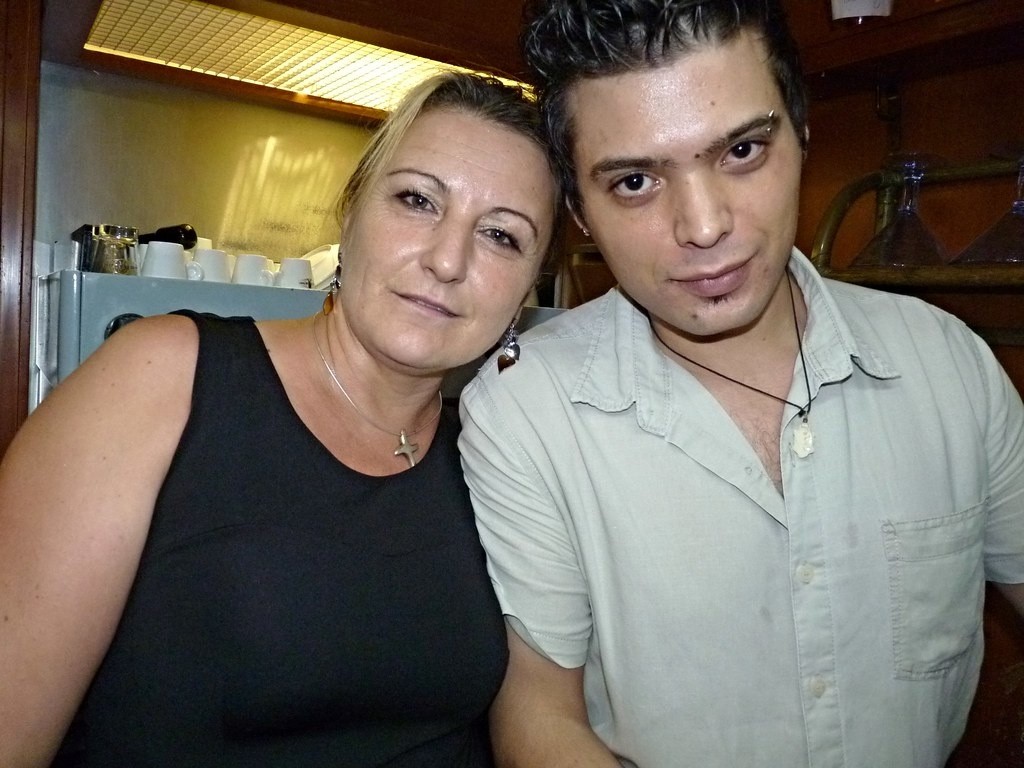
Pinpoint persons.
[0,70,568,768]
[456,0,1024,768]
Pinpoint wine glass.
[946,150,1024,265]
[848,151,952,267]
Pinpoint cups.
[830,0,893,31]
[81,225,313,290]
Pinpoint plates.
[300,244,340,291]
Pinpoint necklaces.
[652,269,814,458]
[313,311,443,468]
